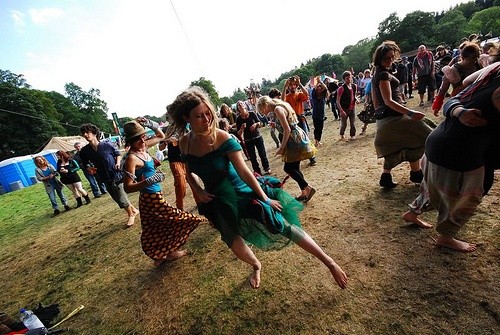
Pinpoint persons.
[73,142,108,200]
[78,122,139,228]
[164,84,348,292]
[55,150,93,209]
[373,32,499,253]
[34,155,72,219]
[164,112,191,211]
[217,62,374,203]
[156,141,168,163]
[123,116,205,267]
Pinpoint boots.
[379,173,397,188]
[82,194,91,205]
[74,197,83,208]
[410,170,424,183]
[243,149,250,160]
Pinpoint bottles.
[0,313,18,328]
[86,160,96,173]
[0,322,10,333]
[19,309,46,331]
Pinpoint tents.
[42,136,89,156]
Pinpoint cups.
[61,167,64,170]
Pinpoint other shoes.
[64,205,71,211]
[51,209,60,219]
[310,155,318,166]
[295,187,316,203]
[95,192,107,197]
[404,91,451,107]
[265,168,272,174]
